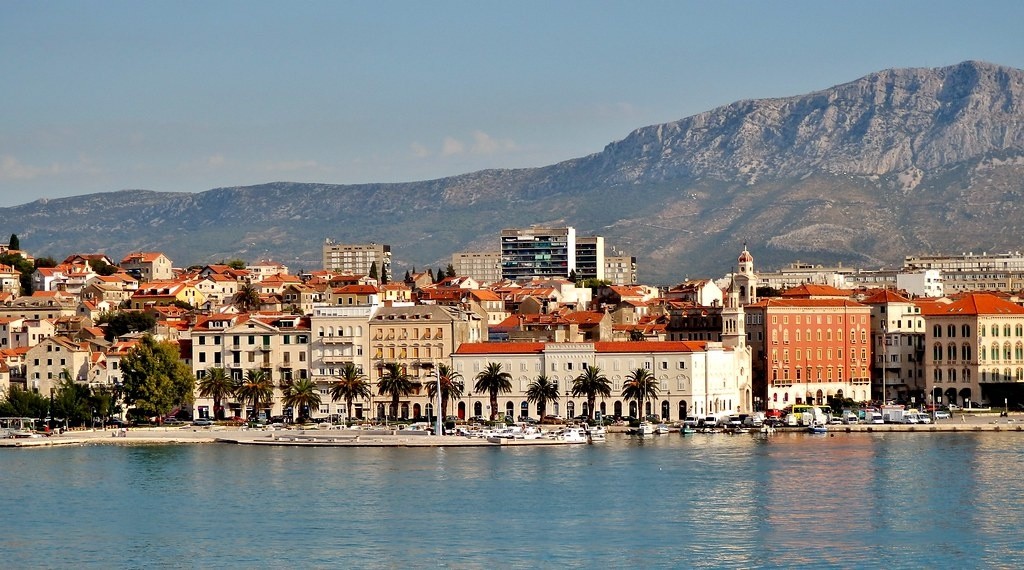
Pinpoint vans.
[288,421,348,430]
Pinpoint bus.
[0,416,67,438]
[792,404,833,426]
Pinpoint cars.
[193,417,213,425]
[164,417,184,424]
[107,417,125,427]
[935,409,951,420]
[931,411,950,420]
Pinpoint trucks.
[832,408,931,425]
[684,409,783,427]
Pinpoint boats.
[494,426,604,440]
